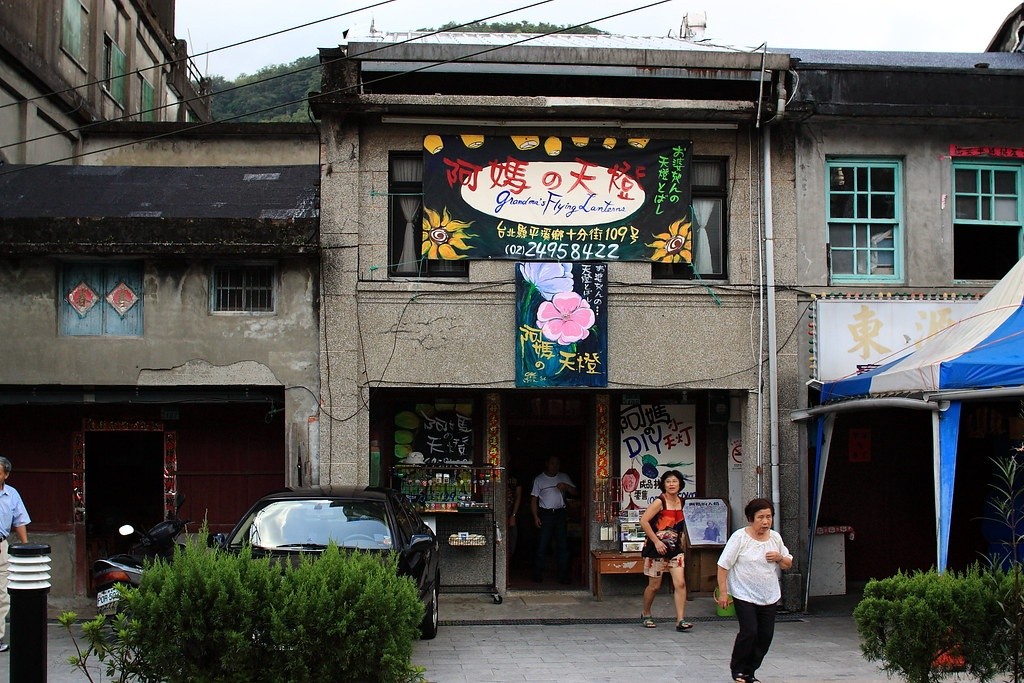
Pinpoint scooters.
[84,493,191,644]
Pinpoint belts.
[0,535,7,542]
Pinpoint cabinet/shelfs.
[391,461,502,603]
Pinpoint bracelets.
[512,513,518,518]
[776,555,783,563]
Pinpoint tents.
[800,254,1024,621]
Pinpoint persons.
[717,498,793,683]
[529,457,578,584]
[0,454,29,652]
[640,470,695,632]
[703,521,722,543]
[506,457,523,578]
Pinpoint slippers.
[641,614,657,628]
[676,620,693,630]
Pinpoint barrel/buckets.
[713,585,737,617]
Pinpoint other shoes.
[731,670,761,683]
[535,573,543,583]
[0,642,9,652]
[559,577,572,585]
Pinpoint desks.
[591,550,674,602]
[808,526,855,596]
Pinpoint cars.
[208,487,443,640]
